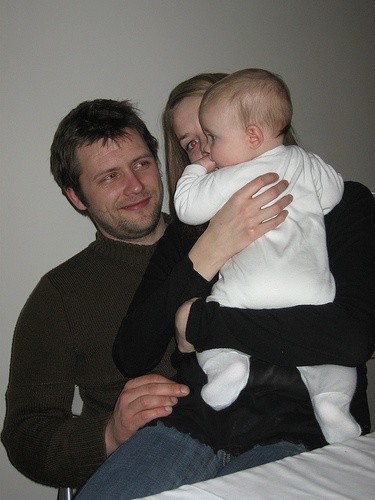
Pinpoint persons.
[70,69,375,500]
[0,95,183,494]
[173,66,362,445]
